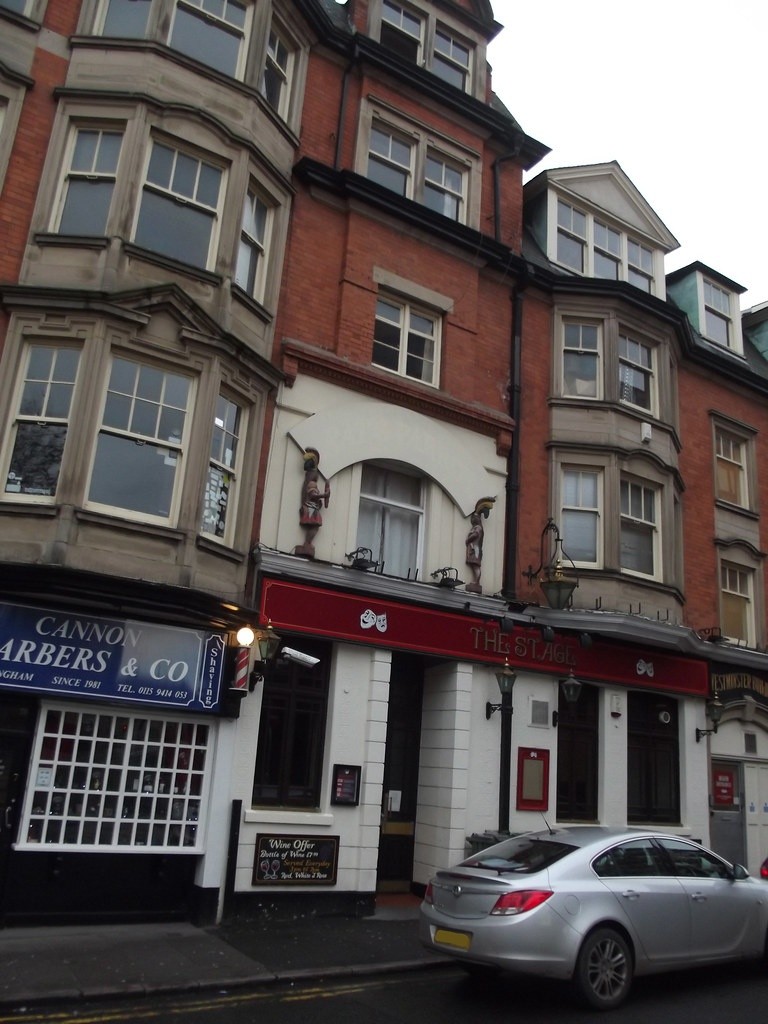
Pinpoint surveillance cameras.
[281,647,321,668]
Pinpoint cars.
[421,827,768,1010]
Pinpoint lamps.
[699,627,729,644]
[229,628,255,694]
[279,647,321,669]
[249,618,282,689]
[486,656,519,722]
[551,669,582,728]
[430,567,466,589]
[345,546,380,571]
[522,517,580,609]
[695,691,725,743]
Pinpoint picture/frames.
[515,746,551,813]
[330,763,362,806]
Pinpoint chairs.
[618,847,651,875]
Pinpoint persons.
[298,447,332,550]
[465,496,495,586]
